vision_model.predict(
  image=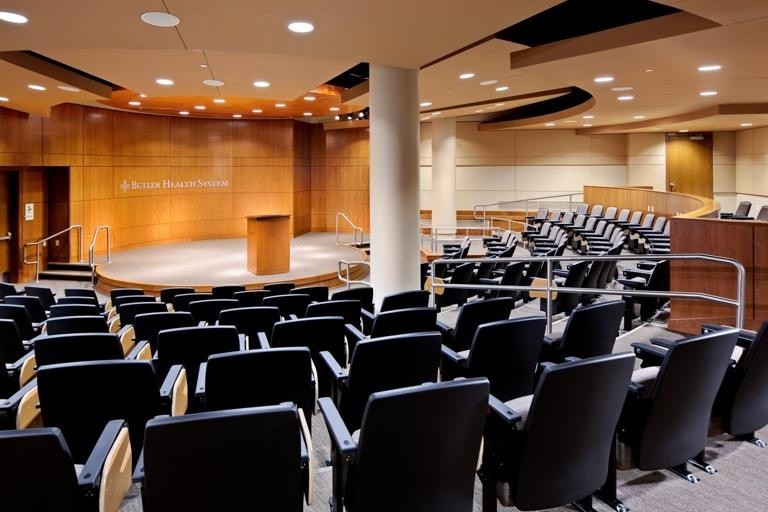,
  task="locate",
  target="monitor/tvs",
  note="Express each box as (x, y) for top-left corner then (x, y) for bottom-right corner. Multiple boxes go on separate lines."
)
(731, 216), (755, 219)
(720, 212), (733, 219)
